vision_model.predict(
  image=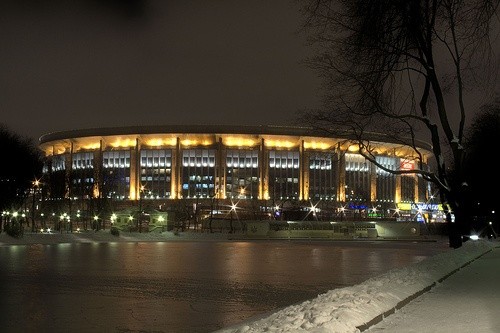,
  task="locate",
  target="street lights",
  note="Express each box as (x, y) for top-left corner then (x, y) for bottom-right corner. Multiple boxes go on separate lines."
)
(3, 195), (436, 236)
